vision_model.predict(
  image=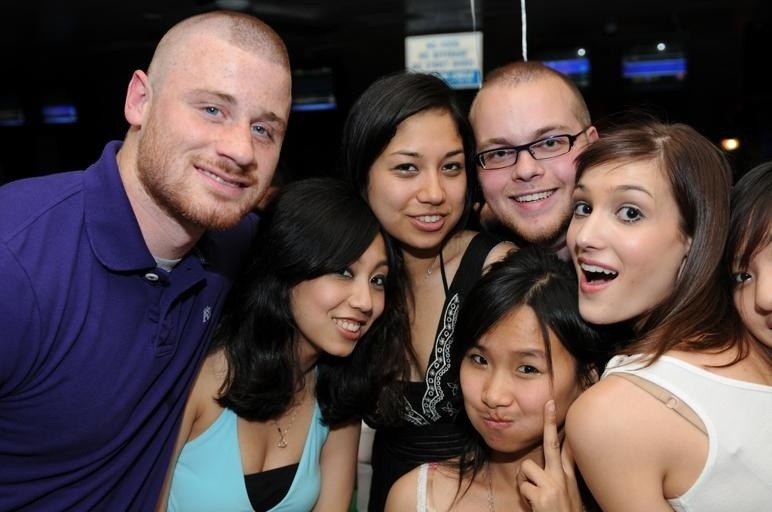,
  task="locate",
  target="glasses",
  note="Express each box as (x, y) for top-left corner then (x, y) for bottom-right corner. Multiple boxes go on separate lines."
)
(475, 128), (588, 170)
